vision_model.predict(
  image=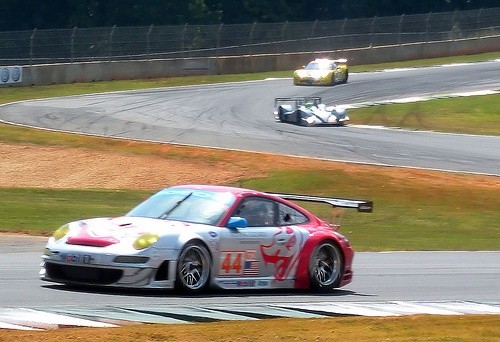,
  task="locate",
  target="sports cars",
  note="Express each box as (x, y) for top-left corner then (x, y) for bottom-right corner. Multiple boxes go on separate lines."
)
(274, 96), (350, 127)
(292, 58), (349, 86)
(40, 185), (373, 294)
